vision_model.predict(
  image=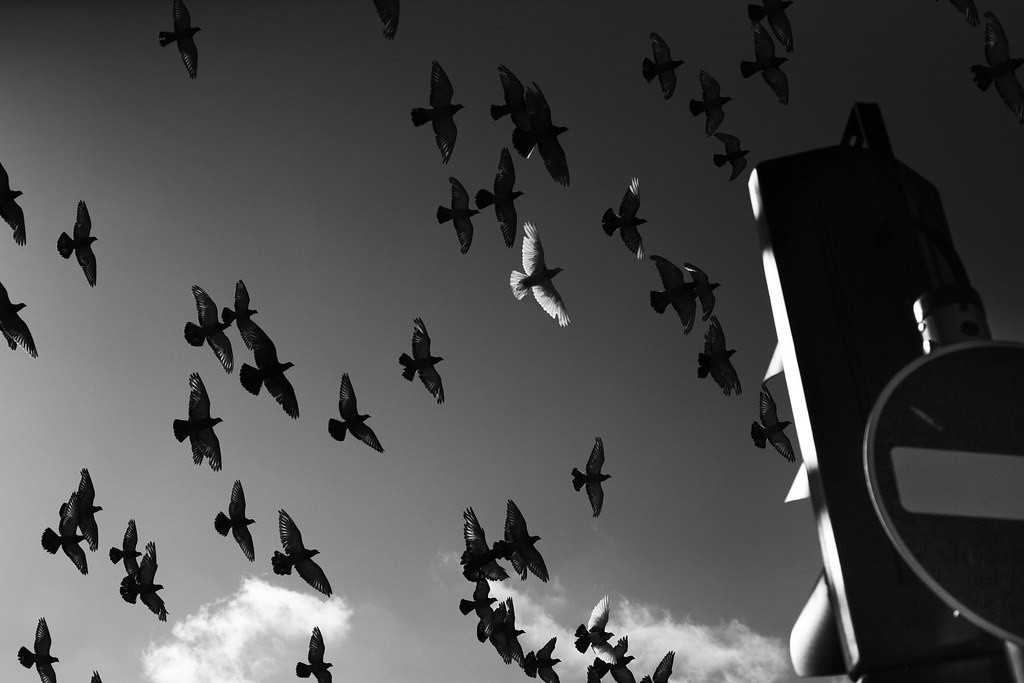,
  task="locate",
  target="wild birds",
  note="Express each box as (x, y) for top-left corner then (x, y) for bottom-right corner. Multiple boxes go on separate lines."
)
(0, 0), (1024, 683)
